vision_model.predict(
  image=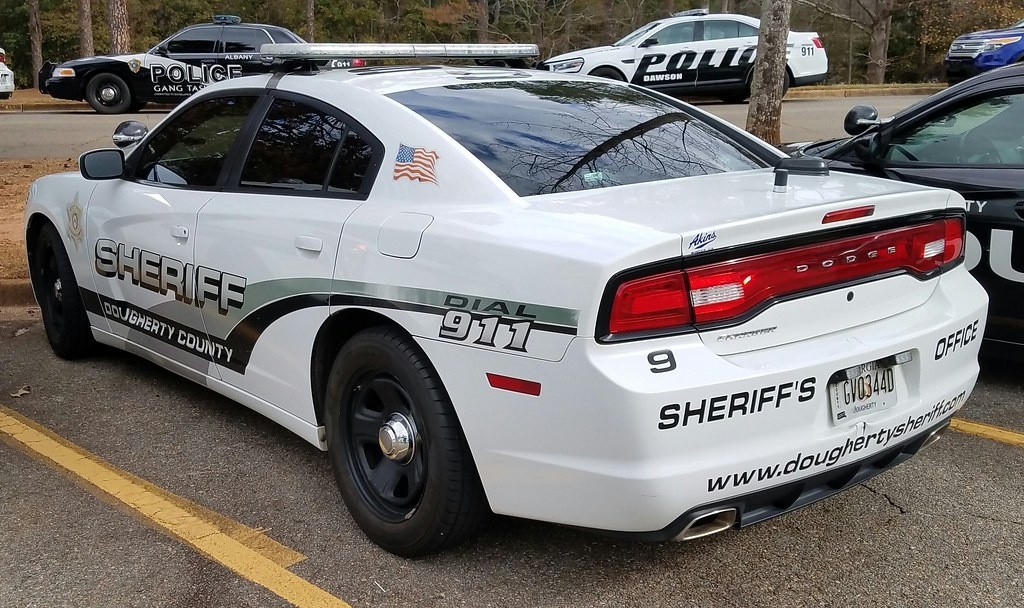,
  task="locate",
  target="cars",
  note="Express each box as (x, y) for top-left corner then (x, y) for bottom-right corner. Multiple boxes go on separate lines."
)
(536, 8), (830, 103)
(941, 20), (1024, 88)
(761, 56), (1024, 382)
(22, 41), (988, 563)
(0, 47), (16, 98)
(37, 13), (367, 117)
